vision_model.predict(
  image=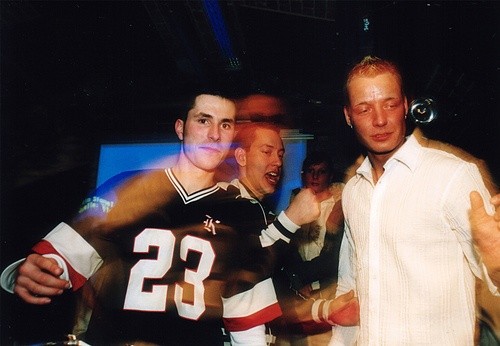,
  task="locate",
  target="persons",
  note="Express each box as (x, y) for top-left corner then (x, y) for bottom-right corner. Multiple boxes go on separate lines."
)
(329, 56), (500, 346)
(0, 87), (283, 346)
(214, 120), (360, 346)
(286, 152), (345, 298)
